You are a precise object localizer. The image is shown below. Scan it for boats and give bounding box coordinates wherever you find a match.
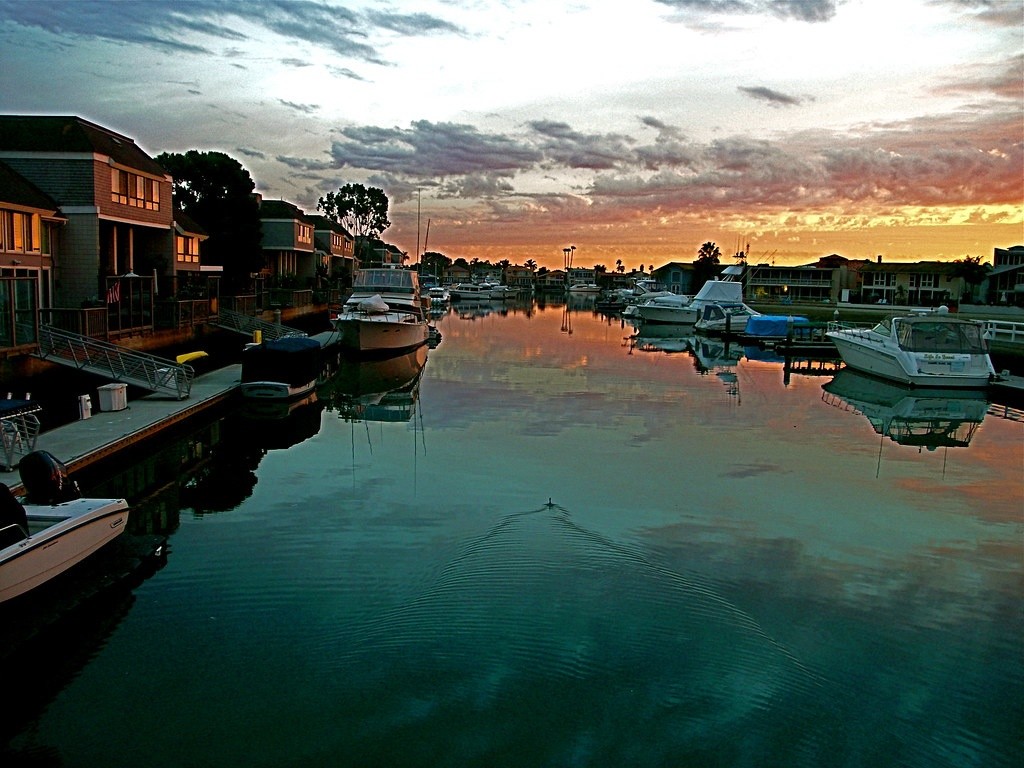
[239,336,325,399]
[820,366,992,480]
[567,283,603,292]
[427,286,451,306]
[329,268,432,353]
[449,280,523,300]
[822,309,998,387]
[320,343,430,422]
[629,322,745,373]
[693,301,764,334]
[594,274,744,324]
[0,449,131,606]
